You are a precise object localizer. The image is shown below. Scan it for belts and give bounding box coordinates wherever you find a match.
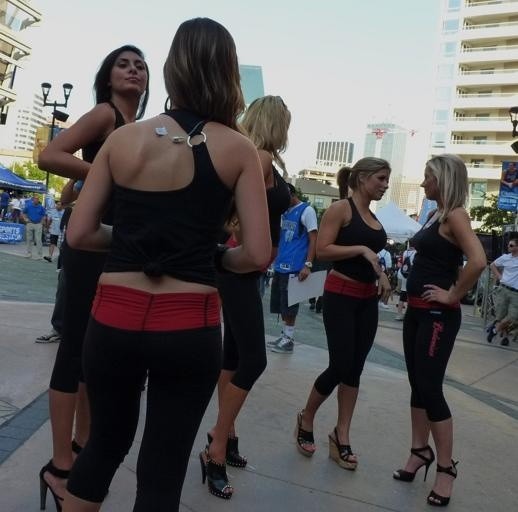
[501,283,518,293]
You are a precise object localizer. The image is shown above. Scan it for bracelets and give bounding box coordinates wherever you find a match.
[215,243,229,274]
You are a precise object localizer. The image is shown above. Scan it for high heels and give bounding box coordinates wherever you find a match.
[206,432,247,467]
[426,458,460,506]
[70,438,85,459]
[392,445,435,481]
[294,409,315,456]
[328,427,359,470]
[39,458,73,512]
[198,443,233,499]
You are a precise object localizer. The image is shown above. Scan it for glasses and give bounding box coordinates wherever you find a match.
[508,243,516,247]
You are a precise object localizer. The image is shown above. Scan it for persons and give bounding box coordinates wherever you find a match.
[293,157,393,470]
[226,232,418,322]
[486,238,518,347]
[265,184,318,353]
[499,162,518,194]
[199,96,291,500]
[36,45,151,510]
[34,178,82,345]
[390,154,489,505]
[0,188,67,264]
[60,16,272,511]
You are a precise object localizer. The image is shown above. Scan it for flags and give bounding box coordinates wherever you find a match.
[497,160,517,214]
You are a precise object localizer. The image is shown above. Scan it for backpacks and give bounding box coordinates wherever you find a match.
[401,250,413,278]
[378,250,388,273]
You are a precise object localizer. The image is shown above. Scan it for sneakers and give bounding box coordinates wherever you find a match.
[487,326,496,343]
[501,337,509,346]
[271,337,294,353]
[43,255,53,262]
[35,330,61,343]
[266,334,284,348]
[37,254,42,260]
[378,300,406,321]
[24,253,32,258]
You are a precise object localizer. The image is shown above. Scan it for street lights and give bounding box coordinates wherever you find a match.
[38,78,75,195]
